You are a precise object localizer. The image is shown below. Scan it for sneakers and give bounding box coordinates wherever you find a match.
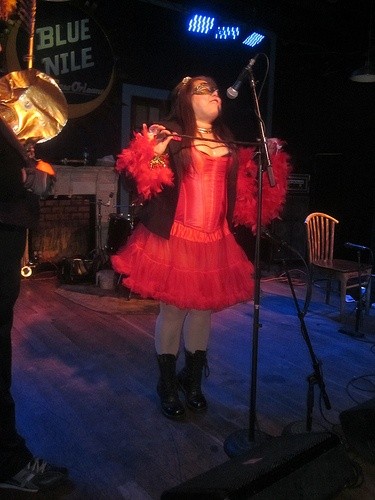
[0,454,69,493]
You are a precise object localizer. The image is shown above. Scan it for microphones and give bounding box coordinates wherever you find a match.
[226,53,262,99]
[345,242,369,251]
[262,226,287,247]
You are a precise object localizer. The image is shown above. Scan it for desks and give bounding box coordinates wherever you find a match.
[20,167,118,273]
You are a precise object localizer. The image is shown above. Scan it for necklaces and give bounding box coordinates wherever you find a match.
[196,126,213,134]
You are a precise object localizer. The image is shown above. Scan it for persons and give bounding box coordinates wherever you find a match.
[110,76,291,419]
[1,117,70,495]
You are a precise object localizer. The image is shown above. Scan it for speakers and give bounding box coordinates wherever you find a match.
[161,432,353,500]
[338,395,375,460]
[262,195,309,262]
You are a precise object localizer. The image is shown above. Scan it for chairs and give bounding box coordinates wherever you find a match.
[299,211,374,326]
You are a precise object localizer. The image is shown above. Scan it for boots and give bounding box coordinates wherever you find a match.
[179,348,210,412]
[155,353,186,418]
